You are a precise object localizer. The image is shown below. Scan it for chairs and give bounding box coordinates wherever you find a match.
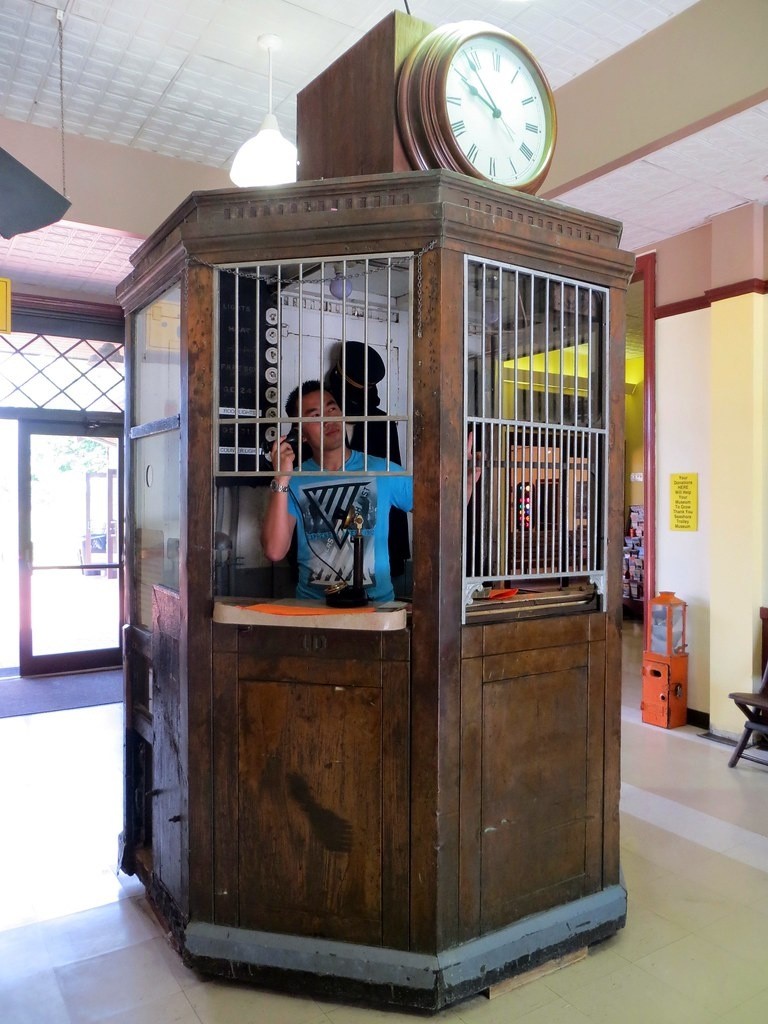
[727,663,768,770]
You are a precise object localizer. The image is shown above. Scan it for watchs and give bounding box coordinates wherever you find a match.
[270,480,288,492]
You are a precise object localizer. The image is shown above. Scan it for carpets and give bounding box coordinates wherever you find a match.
[0,665,124,718]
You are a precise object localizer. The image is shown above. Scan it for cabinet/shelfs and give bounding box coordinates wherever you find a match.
[621,504,644,623]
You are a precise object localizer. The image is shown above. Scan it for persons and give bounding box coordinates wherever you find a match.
[259,380,486,605]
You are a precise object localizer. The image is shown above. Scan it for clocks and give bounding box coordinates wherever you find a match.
[396,20,557,197]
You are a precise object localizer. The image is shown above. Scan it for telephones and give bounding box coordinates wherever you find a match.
[263,428,302,455]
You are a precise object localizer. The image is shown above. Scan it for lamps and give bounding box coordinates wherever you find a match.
[647,591,685,655]
[231,35,297,188]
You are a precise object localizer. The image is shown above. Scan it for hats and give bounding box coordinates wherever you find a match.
[330,342,385,403]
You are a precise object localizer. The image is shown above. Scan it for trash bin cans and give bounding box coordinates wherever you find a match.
[641,592,690,729]
